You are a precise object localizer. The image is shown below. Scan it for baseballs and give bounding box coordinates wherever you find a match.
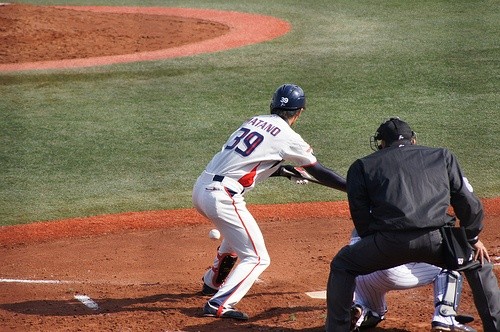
[208,228,221,240]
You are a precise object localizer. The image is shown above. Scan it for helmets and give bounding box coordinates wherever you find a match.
[271,84,305,110]
[374,118,416,142]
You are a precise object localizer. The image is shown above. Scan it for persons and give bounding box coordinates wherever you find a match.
[193,84,348,320]
[326,118,500,332]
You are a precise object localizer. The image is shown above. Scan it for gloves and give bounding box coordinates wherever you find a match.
[291,176,308,184]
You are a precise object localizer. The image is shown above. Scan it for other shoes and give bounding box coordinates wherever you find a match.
[431,320,476,332]
[202,301,249,320]
[351,304,363,332]
[202,287,219,296]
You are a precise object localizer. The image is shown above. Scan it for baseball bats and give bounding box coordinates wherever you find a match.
[279,168,347,192]
